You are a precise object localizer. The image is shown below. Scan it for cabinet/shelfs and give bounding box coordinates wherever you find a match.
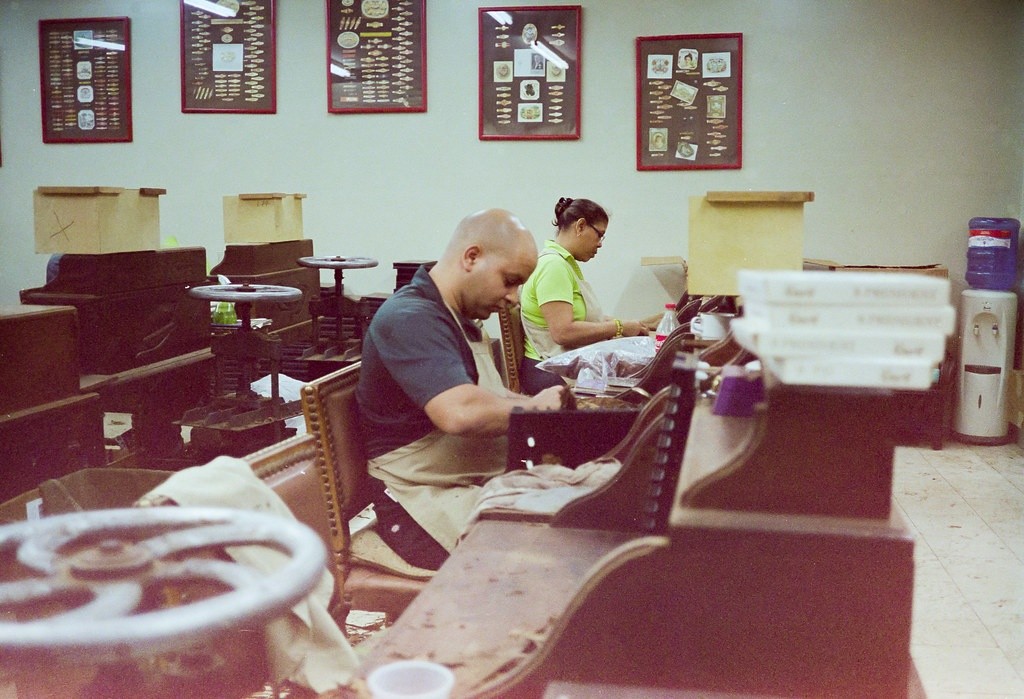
[358,291,930,698]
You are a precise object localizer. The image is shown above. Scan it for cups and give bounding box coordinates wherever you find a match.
[691,313,735,338]
[368,661,454,699]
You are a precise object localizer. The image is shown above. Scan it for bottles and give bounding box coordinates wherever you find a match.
[213,301,237,335]
[655,304,682,356]
[966,217,1020,290]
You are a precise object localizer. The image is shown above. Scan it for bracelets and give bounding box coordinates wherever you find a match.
[615,320,623,336]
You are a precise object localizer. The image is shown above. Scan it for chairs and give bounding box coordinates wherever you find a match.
[299,361,430,623]
[499,296,527,395]
[239,432,430,649]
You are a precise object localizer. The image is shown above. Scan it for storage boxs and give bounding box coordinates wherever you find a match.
[803,258,844,270]
[835,263,948,279]
[687,191,814,296]
[223,192,308,243]
[33,187,167,253]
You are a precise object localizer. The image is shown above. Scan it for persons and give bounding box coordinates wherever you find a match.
[355,210,564,572]
[520,197,650,396]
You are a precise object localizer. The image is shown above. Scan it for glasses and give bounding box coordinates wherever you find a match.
[586,222,606,244]
